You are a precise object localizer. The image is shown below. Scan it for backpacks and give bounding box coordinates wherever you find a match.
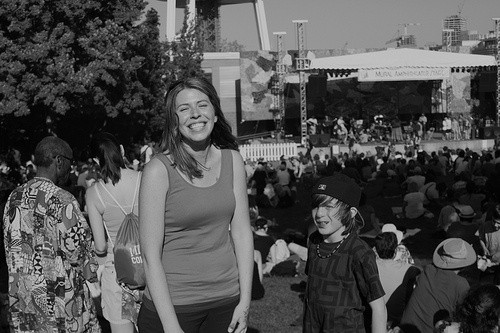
[96,168,146,290]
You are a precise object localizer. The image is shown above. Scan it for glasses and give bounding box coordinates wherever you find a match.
[52,153,76,166]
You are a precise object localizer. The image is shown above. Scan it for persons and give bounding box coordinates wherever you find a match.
[1,76,500,333]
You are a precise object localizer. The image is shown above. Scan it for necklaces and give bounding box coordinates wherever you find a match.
[316,230,349,258]
[34,176,54,185]
[194,148,210,170]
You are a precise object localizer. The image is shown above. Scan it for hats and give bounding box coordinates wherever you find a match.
[313,175,365,228]
[381,224,403,245]
[432,237,478,271]
